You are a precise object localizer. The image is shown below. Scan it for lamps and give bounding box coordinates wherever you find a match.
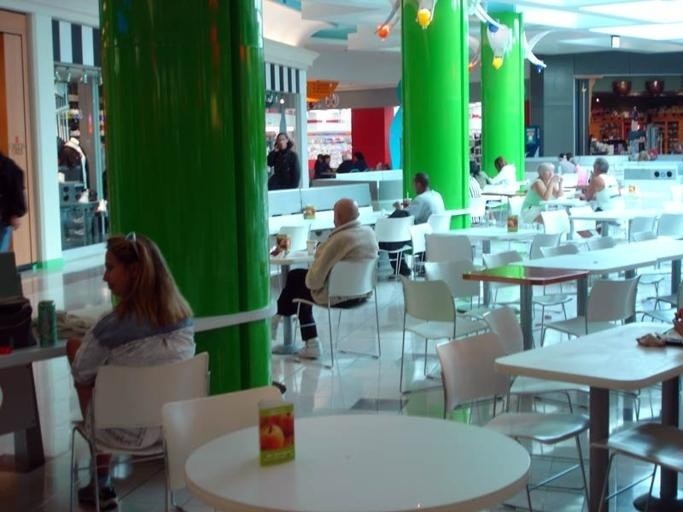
[611,35,621,49]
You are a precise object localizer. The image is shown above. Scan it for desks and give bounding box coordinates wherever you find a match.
[0,305,111,473]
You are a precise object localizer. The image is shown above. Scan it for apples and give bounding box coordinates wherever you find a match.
[260,424,285,450]
[276,412,295,436]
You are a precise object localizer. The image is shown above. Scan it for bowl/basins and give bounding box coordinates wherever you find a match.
[644,80,664,97]
[612,80,631,96]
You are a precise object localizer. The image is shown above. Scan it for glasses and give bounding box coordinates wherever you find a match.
[123,230,139,261]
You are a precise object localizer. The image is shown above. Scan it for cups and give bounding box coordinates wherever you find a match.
[306,239,318,256]
[403,198,411,208]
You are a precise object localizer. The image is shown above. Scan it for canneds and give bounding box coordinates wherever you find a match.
[38,300,58,349]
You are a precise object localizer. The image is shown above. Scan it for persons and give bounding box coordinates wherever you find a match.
[388,172,447,282]
[467,117,648,240]
[312,149,391,178]
[667,140,683,154]
[0,151,26,251]
[67,231,198,512]
[267,131,302,190]
[270,197,379,358]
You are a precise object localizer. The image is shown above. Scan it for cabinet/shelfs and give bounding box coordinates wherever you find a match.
[651,114,683,154]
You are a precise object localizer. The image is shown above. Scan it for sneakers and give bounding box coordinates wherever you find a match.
[294,340,323,359]
[382,268,413,281]
[77,482,118,511]
